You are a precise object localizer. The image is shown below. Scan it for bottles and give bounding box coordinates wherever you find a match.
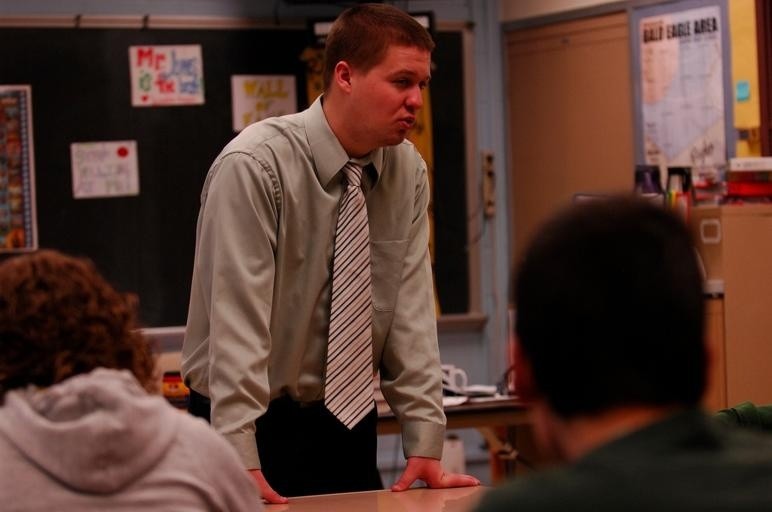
[666,164,696,207]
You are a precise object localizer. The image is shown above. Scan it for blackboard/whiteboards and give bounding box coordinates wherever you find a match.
[0,20,487,348]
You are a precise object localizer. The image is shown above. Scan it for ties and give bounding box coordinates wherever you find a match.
[324,163,375,431]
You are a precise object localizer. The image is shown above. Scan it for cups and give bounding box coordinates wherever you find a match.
[440,362,468,388]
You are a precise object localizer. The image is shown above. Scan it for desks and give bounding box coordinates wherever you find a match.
[252,390,551,512]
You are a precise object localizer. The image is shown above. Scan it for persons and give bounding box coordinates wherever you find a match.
[179,0,481,503]
[464,191,772,512]
[0,249,267,512]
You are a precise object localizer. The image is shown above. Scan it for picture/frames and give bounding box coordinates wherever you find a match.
[2,85,44,257]
